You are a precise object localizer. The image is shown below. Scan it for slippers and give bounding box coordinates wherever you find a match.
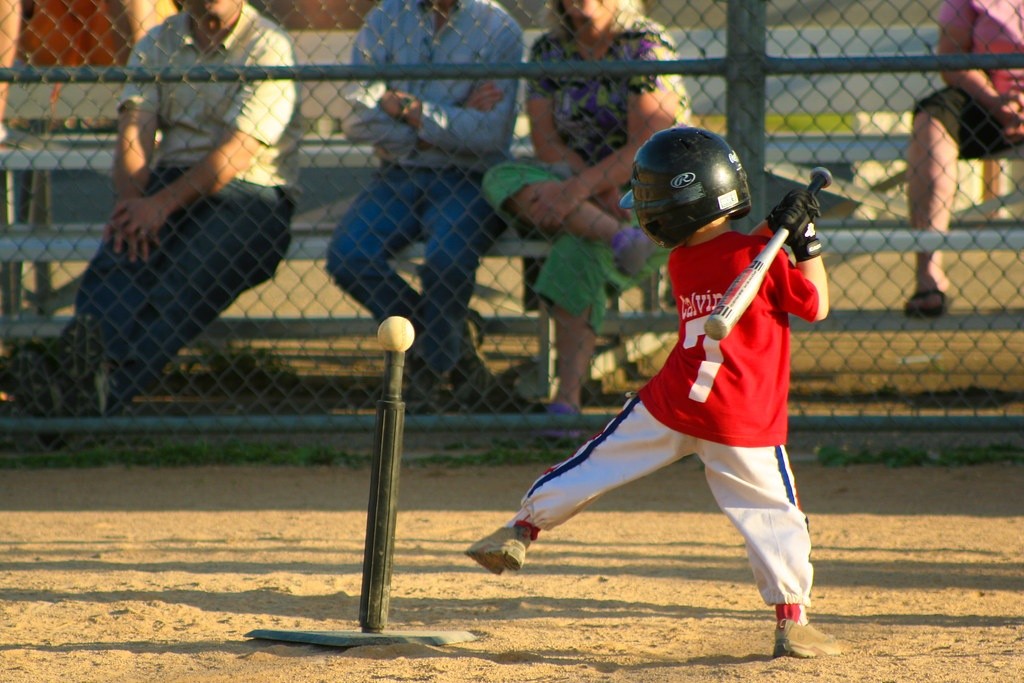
[904,290,946,317]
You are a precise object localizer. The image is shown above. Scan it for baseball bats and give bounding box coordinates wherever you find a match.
[703,166,835,342]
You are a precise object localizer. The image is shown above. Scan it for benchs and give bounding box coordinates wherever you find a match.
[0,24,1024,410]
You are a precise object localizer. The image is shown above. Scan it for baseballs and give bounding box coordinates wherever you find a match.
[376,315,415,352]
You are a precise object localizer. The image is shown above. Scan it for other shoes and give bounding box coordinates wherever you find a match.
[14,336,66,449]
[533,402,581,439]
[614,229,653,270]
[61,313,108,420]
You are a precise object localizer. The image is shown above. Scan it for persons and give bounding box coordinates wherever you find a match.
[0,0,297,448]
[326,0,857,660]
[904,0,1024,316]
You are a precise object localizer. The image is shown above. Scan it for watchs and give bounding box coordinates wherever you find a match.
[396,98,412,120]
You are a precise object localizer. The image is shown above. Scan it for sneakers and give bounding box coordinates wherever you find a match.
[466,526,533,574]
[772,618,844,659]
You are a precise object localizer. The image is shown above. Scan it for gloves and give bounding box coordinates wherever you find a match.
[766,187,824,262]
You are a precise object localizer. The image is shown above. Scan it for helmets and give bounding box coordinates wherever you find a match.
[620,126,750,248]
